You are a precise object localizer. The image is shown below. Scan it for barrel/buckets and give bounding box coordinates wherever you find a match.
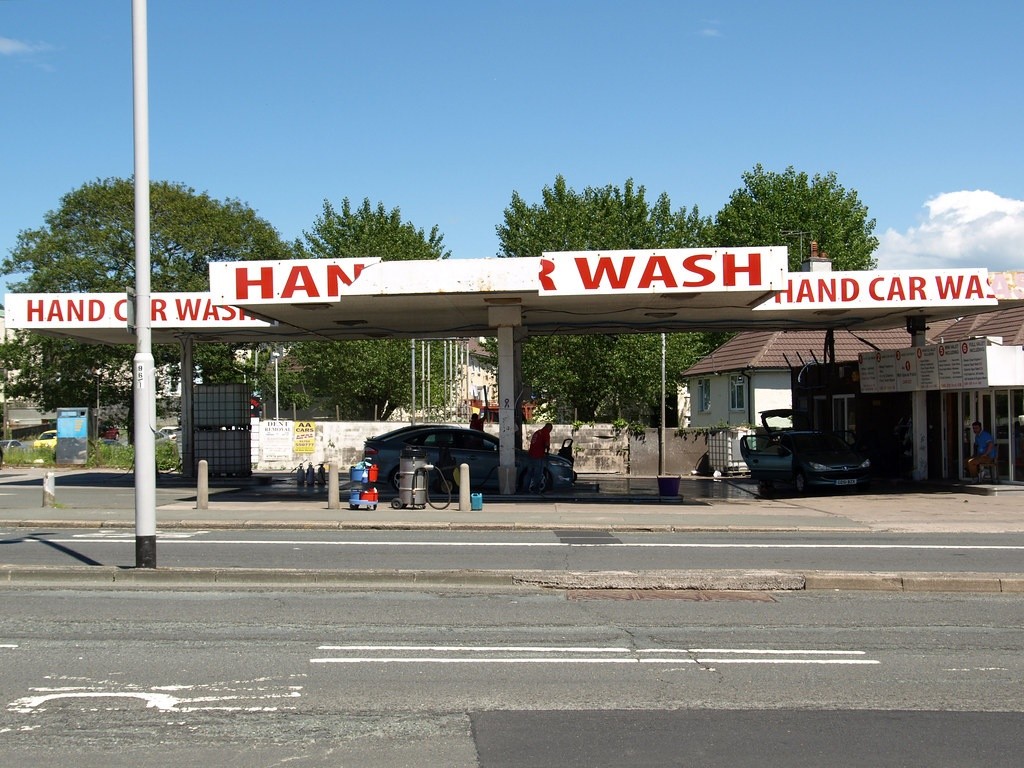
[656,476,681,496]
[471,493,482,510]
[350,468,379,501]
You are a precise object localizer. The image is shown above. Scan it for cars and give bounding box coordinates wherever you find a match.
[34,430,57,450]
[97,426,120,441]
[0,440,29,455]
[740,409,873,495]
[154,426,179,448]
[363,424,574,496]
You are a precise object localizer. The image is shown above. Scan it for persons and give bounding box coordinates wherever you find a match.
[523,423,552,494]
[968,421,997,485]
[470,406,489,431]
